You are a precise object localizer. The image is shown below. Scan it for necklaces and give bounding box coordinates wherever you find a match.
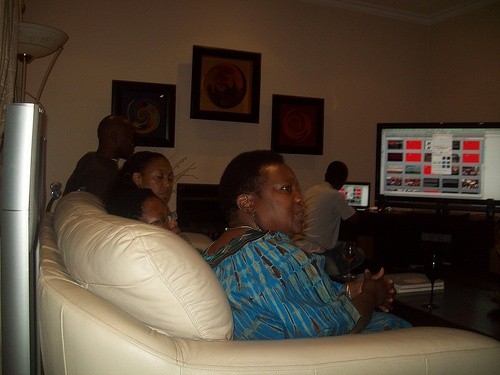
[224,225,262,233]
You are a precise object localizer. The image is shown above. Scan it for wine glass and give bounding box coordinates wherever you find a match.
[420,251,444,311]
[339,239,359,280]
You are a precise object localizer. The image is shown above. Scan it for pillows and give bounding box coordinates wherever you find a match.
[54,192,232,341]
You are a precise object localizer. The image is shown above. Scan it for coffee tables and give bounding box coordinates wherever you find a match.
[393,293,500,342]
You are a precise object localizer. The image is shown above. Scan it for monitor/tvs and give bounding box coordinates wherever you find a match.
[337,181,371,211]
[375,121,500,213]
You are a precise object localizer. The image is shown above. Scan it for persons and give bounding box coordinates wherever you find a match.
[198,149,414,340]
[60,114,414,340]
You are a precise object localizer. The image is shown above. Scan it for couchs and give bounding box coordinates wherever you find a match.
[35,191,500,375]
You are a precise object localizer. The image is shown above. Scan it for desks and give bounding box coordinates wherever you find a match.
[325,204,499,291]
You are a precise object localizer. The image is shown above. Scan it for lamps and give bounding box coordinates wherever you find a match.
[15,23,70,114]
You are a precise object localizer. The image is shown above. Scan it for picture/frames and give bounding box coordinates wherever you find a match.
[110,79,176,149]
[190,44,262,123]
[270,93,325,155]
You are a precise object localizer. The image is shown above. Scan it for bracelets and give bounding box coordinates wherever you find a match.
[345,283,353,301]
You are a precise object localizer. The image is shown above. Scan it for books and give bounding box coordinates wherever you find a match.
[385,274,444,293]
